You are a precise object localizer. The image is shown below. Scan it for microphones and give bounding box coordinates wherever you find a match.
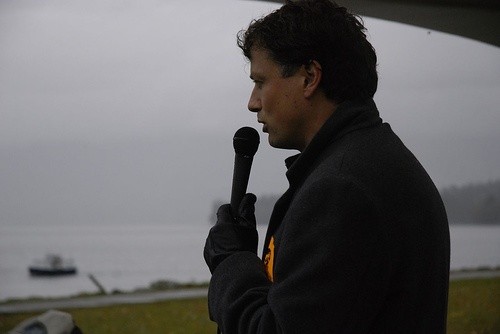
[230,126,261,219]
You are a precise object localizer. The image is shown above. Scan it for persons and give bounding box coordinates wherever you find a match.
[203,0,451,334]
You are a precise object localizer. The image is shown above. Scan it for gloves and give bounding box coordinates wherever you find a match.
[203,193,258,275]
